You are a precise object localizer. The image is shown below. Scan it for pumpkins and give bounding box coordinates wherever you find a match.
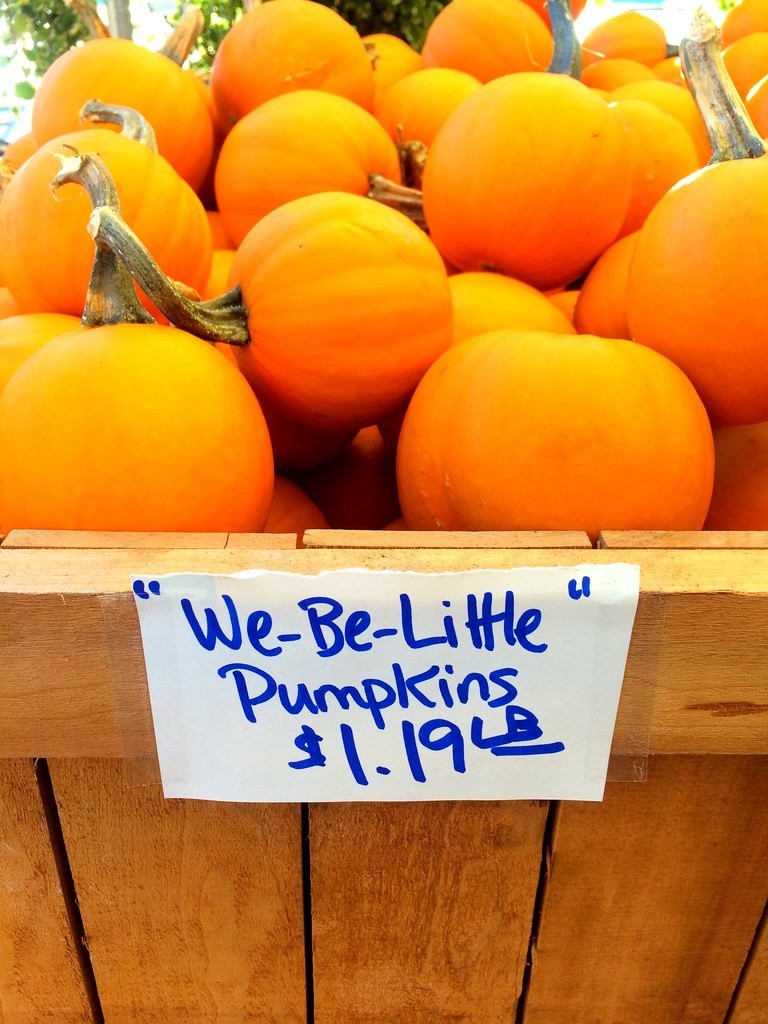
[0,0,768,546]
